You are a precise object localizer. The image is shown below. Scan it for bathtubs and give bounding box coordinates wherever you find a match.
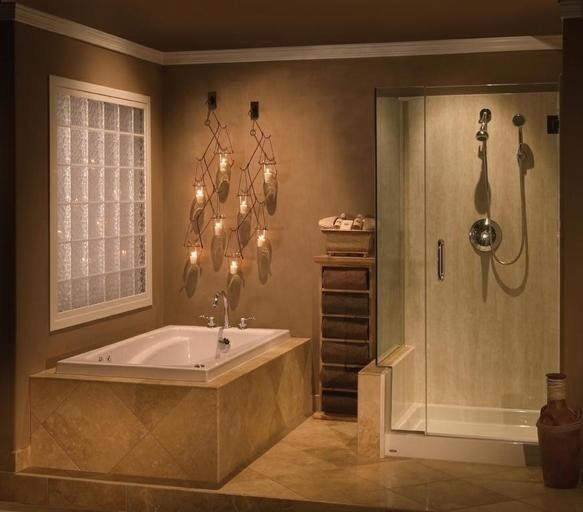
[22,324,315,488]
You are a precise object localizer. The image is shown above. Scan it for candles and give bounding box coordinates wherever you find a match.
[195,189,204,205]
[230,261,238,275]
[219,158,227,172]
[214,222,222,236]
[264,168,272,183]
[257,235,266,247]
[189,251,197,265]
[240,201,248,214]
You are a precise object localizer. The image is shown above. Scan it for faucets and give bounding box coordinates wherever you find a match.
[213,289,231,328]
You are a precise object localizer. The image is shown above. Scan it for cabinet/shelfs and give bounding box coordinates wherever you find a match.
[313,251,376,423]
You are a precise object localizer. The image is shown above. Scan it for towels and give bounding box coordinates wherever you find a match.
[319,269,368,416]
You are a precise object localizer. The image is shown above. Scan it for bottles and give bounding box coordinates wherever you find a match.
[351,213,363,231]
[333,212,345,229]
